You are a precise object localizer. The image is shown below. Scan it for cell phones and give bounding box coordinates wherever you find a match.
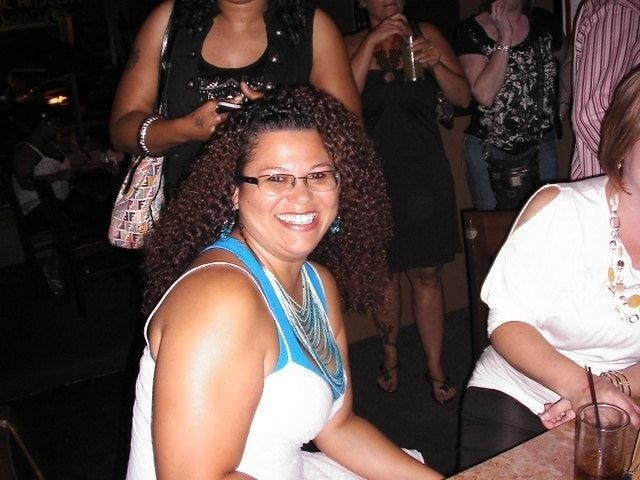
[216,100,243,117]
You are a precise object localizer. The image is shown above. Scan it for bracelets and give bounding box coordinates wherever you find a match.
[137,112,171,159]
[485,44,511,55]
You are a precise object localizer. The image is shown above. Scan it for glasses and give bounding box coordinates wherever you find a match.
[237,169,342,196]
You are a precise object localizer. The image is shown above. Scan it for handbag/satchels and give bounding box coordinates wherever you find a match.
[108,153,169,250]
[486,150,540,210]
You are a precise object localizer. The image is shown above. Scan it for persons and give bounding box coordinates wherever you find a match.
[448,0,576,213]
[107,0,372,210]
[118,81,451,480]
[312,0,474,404]
[563,0,640,183]
[433,57,640,476]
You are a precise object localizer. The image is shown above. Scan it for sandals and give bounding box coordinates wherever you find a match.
[377,361,400,392]
[428,375,455,406]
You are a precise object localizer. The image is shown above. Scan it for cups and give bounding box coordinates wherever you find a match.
[574,403,632,480]
[402,32,426,84]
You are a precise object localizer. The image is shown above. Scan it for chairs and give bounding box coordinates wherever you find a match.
[462,207,520,365]
[0,166,138,316]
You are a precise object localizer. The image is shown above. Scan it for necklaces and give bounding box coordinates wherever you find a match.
[602,186,640,326]
[368,33,405,83]
[235,223,350,396]
